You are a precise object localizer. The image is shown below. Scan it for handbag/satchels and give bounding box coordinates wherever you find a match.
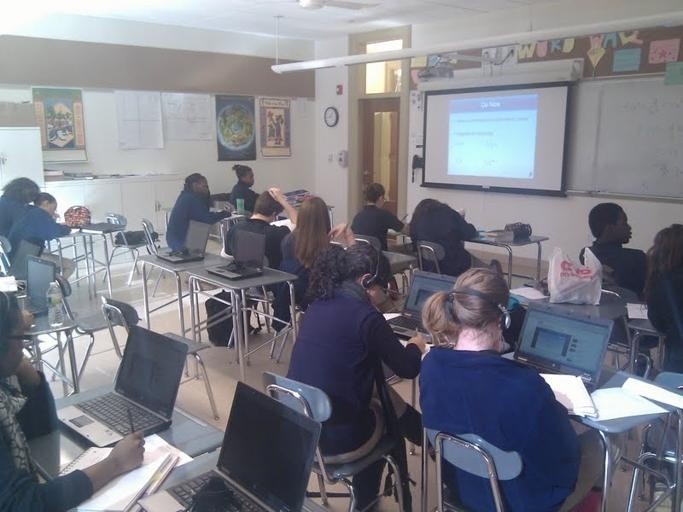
[64,205,91,227]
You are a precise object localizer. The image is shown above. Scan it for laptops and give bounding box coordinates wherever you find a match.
[15,254,57,316]
[513,300,607,384]
[157,219,211,263]
[386,270,458,343]
[205,229,267,280]
[135,380,325,512]
[56,326,191,448]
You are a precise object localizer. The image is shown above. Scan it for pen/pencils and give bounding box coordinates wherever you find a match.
[127,408,135,435]
[415,328,418,337]
[400,214,409,223]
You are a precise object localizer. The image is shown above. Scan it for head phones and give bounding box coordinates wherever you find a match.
[184,477,245,511]
[444,288,512,332]
[168,247,189,257]
[226,260,245,273]
[343,237,381,290]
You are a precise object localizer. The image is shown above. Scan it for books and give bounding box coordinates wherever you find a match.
[541,373,597,418]
[81,222,123,235]
[57,446,169,508]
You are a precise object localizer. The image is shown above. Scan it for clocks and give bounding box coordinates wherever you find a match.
[323,107,339,127]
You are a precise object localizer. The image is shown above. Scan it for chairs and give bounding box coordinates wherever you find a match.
[0,235,12,271]
[626,371,683,511]
[51,272,121,383]
[141,217,177,298]
[14,312,80,391]
[104,295,220,421]
[422,427,523,510]
[103,213,158,286]
[261,369,407,508]
[415,241,445,275]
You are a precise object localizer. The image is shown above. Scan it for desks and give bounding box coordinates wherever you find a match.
[57,223,126,298]
[137,247,233,339]
[186,258,299,381]
[567,366,668,511]
[471,231,550,287]
[357,248,419,274]
[627,319,666,377]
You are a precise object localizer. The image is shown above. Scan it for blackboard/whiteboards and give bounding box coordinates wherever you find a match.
[561,71,682,202]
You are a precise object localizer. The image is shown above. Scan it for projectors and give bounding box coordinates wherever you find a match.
[417,67,454,79]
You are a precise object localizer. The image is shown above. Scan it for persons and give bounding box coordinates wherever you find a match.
[580,203,661,380]
[1,292,145,510]
[646,224,683,373]
[225,187,297,335]
[412,198,503,277]
[230,164,287,221]
[166,173,235,253]
[286,242,436,512]
[0,178,40,237]
[272,196,358,332]
[419,268,624,511]
[352,183,411,300]
[7,192,76,279]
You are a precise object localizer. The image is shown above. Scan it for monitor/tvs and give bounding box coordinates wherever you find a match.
[7,238,42,281]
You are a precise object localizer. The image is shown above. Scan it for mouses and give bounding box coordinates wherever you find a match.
[18,282,25,291]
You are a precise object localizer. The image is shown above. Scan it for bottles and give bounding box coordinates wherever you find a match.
[47,282,64,327]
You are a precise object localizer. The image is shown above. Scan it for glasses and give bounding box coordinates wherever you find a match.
[4,334,33,345]
